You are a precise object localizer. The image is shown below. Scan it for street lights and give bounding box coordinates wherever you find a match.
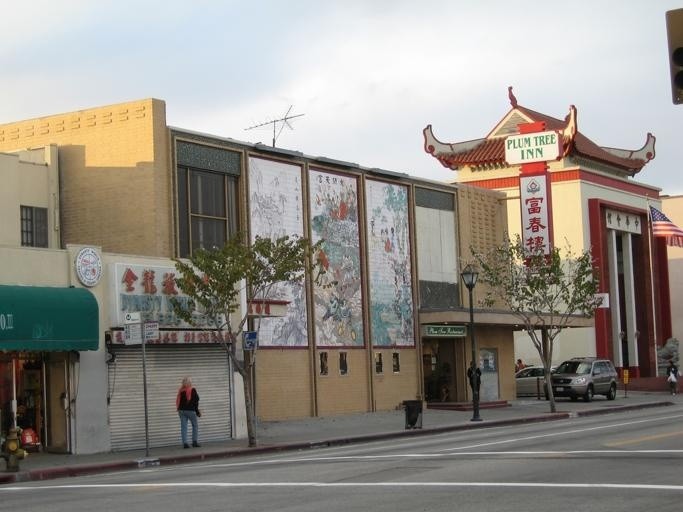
[459,263,483,421]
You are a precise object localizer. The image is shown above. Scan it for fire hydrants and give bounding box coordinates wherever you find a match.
[0,428,32,472]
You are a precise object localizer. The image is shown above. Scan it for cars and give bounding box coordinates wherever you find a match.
[515,356,618,402]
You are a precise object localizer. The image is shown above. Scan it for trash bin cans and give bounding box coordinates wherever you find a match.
[402,399,423,429]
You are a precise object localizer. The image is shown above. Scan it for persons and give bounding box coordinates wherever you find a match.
[466,360,481,402]
[665,360,678,396]
[174,376,202,448]
[516,359,524,370]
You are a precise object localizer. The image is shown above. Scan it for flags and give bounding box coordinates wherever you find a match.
[649,205,683,249]
